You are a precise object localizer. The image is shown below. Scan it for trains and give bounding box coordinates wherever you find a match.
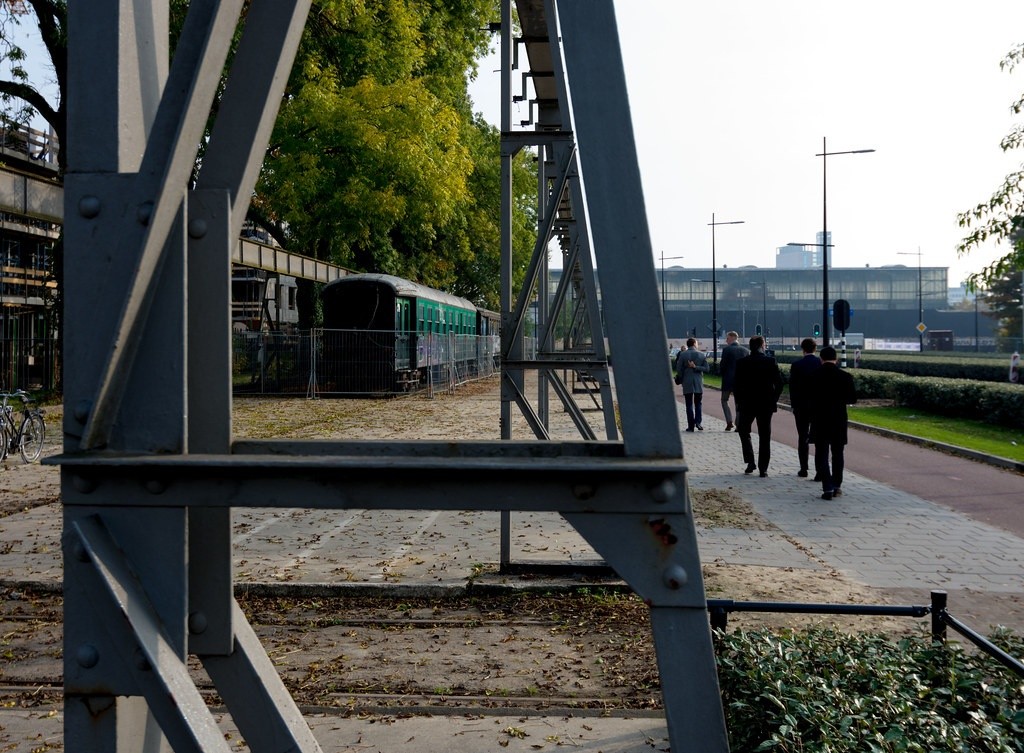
[317,273,502,391]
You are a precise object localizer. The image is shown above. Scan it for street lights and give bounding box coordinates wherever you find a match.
[658,252,684,317]
[689,279,701,340]
[694,213,746,364]
[787,136,878,345]
[748,271,767,350]
[897,246,931,352]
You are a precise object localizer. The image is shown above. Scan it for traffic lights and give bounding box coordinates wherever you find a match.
[691,326,696,338]
[814,322,821,337]
[755,324,762,336]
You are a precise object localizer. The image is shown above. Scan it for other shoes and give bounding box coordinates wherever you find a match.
[833,488,842,497]
[725,423,734,431]
[822,492,834,500]
[760,472,768,477]
[798,470,808,477]
[696,423,703,430]
[814,473,822,481]
[686,427,695,432]
[735,426,739,432]
[745,463,756,473]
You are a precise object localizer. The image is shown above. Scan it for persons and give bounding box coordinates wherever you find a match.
[674,338,709,433]
[804,347,857,500]
[675,345,687,368]
[717,331,749,432]
[789,341,824,479]
[733,336,785,479]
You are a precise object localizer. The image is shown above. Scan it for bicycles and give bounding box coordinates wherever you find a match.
[0,390,47,463]
